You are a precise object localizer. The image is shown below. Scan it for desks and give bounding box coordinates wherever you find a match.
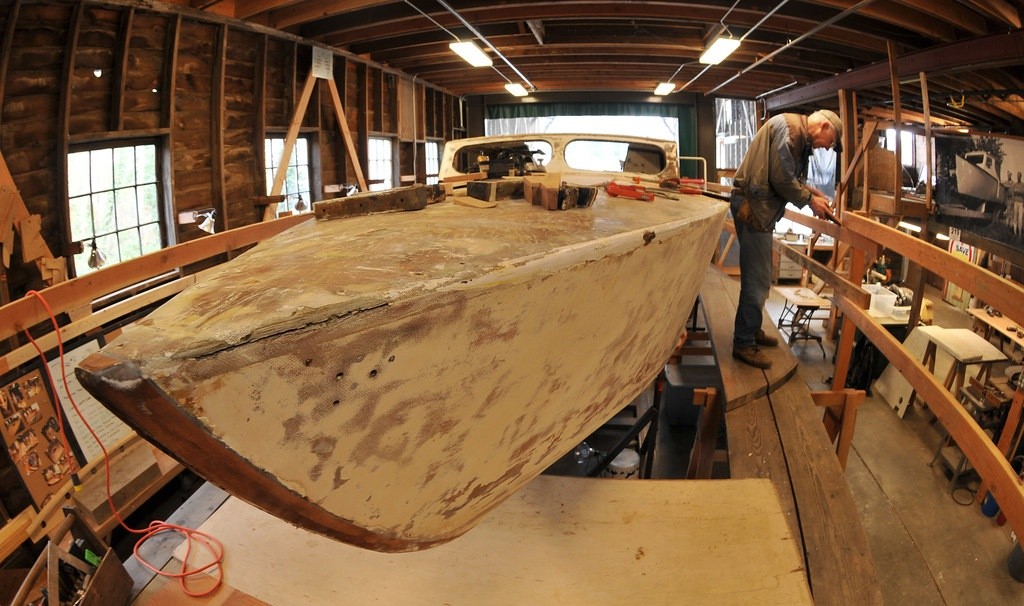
[772,288,827,355]
[966,307,1024,352]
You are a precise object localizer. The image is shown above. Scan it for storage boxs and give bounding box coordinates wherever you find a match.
[887,286,911,320]
[862,283,897,318]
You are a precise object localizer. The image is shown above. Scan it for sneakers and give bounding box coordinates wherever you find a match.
[754,330,778,346]
[732,343,772,368]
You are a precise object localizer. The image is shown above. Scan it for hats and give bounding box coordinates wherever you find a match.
[819,109,843,153]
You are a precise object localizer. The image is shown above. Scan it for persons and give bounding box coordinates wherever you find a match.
[730,110,844,369]
[1,382,77,480]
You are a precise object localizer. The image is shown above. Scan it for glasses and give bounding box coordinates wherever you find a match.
[830,127,837,148]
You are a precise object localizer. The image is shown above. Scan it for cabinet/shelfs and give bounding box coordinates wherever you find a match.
[779,245,805,280]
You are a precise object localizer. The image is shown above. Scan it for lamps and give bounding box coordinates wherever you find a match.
[178,207,215,235]
[293,195,309,210]
[324,183,360,196]
[699,36,742,65]
[505,81,529,97]
[653,81,676,96]
[87,240,106,269]
[450,38,493,68]
